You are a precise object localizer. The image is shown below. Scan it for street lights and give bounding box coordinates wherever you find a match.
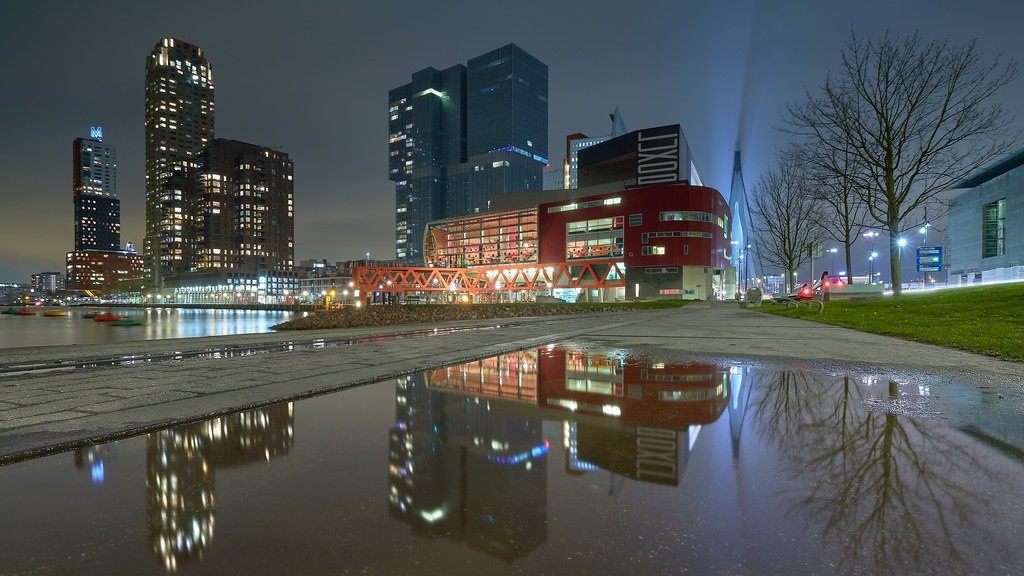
[781,272,787,294]
[763,275,767,294]
[771,275,777,294]
[793,271,798,286]
[863,230,878,285]
[755,277,760,288]
[921,220,931,290]
[743,243,752,292]
[827,247,837,277]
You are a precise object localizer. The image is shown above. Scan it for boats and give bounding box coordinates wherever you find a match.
[109,319,147,326]
[14,308,36,316]
[92,313,128,321]
[2,307,19,314]
[81,311,101,319]
[41,308,70,317]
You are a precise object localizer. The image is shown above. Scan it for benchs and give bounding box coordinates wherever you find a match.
[773,283,807,309]
[788,279,824,314]
